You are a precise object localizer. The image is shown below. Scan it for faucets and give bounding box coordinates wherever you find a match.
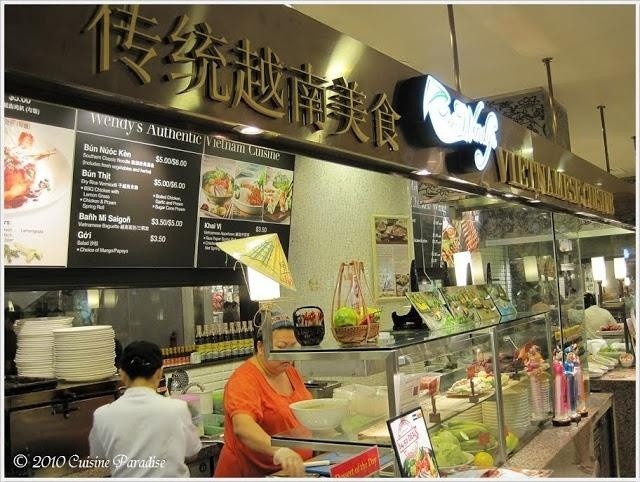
[181,382,204,394]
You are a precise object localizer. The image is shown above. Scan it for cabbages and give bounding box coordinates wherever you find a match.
[431,430,464,467]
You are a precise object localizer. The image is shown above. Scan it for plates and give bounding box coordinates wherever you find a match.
[303,382,328,388]
[450,376,550,437]
[401,362,424,375]
[234,176,262,214]
[12,315,117,384]
[587,356,618,379]
[436,440,499,471]
[4,138,67,214]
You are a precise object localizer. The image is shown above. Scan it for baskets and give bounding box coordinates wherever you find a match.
[332,261,380,344]
[293,306,325,345]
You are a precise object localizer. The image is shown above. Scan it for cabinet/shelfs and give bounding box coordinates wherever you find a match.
[264,308,558,479]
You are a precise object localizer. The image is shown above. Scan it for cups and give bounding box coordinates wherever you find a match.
[620,354,634,367]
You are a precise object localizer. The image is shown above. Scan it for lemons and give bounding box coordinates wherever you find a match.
[474,452,494,468]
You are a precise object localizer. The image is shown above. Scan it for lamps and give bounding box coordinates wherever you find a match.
[613,256,628,297]
[213,232,297,359]
[522,255,540,285]
[451,250,475,288]
[468,249,493,284]
[590,255,607,307]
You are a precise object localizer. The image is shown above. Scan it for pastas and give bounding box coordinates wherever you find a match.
[376,221,407,242]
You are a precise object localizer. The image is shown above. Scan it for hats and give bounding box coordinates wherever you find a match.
[122,342,164,368]
[253,304,294,339]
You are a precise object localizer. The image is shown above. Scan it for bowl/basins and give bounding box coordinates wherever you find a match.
[202,414,225,435]
[396,274,409,286]
[448,227,457,239]
[386,225,407,240]
[287,398,349,432]
[201,171,235,206]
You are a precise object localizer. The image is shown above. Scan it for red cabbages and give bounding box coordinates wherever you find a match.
[333,307,358,328]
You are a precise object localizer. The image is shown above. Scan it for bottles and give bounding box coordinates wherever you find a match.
[161,345,197,368]
[553,358,586,423]
[194,320,254,363]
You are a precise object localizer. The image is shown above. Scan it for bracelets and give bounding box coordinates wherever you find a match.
[273,447,288,466]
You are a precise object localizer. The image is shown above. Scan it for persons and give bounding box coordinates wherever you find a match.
[530,294,549,311]
[88,340,204,477]
[212,311,342,477]
[565,293,618,340]
